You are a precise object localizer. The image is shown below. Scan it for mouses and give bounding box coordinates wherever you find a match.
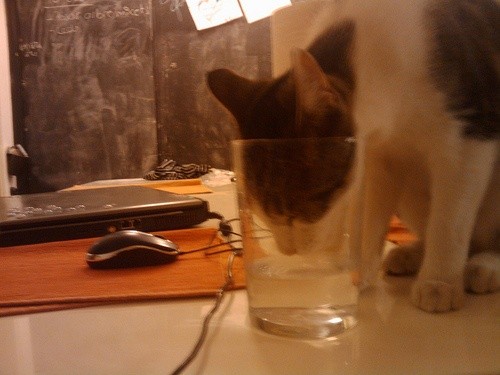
[87,229,180,268]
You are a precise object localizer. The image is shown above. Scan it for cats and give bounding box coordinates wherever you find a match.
[205,0,500,312]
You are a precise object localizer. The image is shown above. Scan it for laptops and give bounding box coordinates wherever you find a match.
[0,184,211,248]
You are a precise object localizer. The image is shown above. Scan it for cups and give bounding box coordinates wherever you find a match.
[229,133,371,342]
